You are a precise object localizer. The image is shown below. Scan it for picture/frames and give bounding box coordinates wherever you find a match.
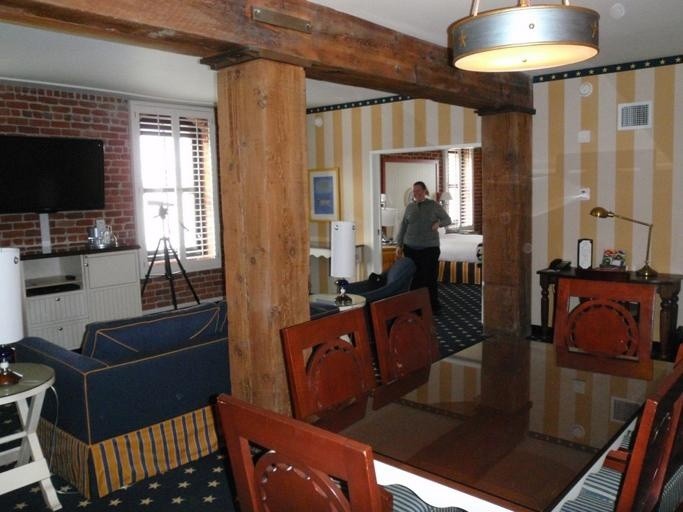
[307,166,341,223]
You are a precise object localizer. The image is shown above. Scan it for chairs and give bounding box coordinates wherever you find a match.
[552,361,683,512]
[606,343,683,512]
[371,286,441,386]
[552,277,655,360]
[280,306,376,421]
[215,391,462,512]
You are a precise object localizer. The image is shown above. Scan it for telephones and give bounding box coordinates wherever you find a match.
[549,258,571,270]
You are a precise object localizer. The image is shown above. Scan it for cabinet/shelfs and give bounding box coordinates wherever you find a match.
[20,244,143,351]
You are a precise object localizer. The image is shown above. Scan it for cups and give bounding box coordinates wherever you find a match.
[88,237,95,250]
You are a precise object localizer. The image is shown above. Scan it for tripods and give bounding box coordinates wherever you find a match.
[141,204,203,311]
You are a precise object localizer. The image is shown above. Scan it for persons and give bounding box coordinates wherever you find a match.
[395,181,452,316]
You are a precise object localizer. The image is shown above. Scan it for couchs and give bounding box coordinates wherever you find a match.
[346,256,416,302]
[14,298,340,500]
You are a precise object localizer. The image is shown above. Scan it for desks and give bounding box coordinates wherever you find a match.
[382,240,397,273]
[536,265,683,362]
[301,333,676,512]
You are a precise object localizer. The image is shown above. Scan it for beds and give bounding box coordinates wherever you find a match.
[438,226,483,286]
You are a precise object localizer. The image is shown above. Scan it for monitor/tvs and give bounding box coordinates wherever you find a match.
[0,132,105,216]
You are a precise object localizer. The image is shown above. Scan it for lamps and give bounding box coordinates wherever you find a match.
[329,220,356,307]
[439,191,452,208]
[381,202,397,228]
[0,247,26,386]
[448,0,601,75]
[589,206,658,278]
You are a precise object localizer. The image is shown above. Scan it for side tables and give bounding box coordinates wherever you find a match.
[0,361,63,511]
